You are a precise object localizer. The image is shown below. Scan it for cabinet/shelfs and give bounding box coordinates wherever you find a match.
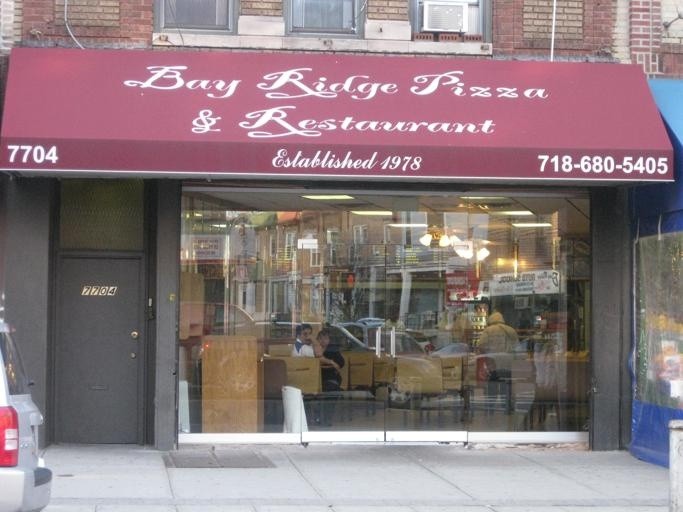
[199,334,264,432]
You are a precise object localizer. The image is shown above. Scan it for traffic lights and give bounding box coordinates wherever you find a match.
[342,272,355,290]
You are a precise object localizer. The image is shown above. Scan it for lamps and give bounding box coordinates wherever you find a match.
[416,212,462,246]
[451,211,490,261]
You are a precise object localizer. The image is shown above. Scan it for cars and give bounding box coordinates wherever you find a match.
[473,335,552,383]
[336,317,430,359]
[192,321,443,409]
[428,341,483,398]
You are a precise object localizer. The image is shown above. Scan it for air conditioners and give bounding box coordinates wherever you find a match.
[417,1,468,41]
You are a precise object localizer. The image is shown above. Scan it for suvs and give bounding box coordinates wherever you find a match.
[0,321,52,512]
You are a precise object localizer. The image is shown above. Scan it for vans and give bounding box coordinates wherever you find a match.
[178,300,252,373]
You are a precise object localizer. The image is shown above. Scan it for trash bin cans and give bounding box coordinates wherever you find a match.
[667,419,683,512]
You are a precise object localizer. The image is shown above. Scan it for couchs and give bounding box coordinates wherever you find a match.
[264,342,592,430]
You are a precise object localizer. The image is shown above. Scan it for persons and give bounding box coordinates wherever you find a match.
[478,311,520,416]
[382,306,404,352]
[290,324,323,358]
[313,330,345,426]
